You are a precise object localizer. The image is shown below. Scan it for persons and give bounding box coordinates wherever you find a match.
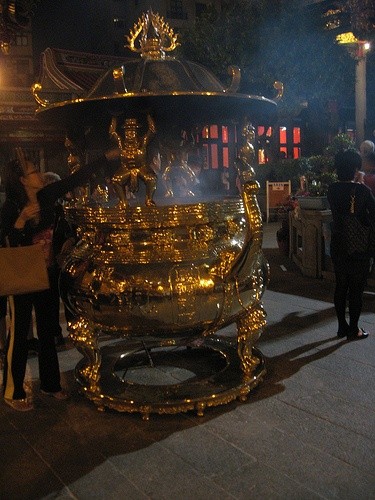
[328,148,375,341]
[359,140,375,197]
[108,105,198,210]
[231,115,256,199]
[0,150,231,413]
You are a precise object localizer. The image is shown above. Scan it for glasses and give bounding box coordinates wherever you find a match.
[27,168,39,175]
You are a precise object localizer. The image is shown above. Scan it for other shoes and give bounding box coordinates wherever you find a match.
[4,398,33,411]
[40,390,68,400]
[347,328,368,340]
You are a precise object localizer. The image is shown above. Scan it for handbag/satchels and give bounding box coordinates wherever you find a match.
[0,236,50,297]
[340,182,375,258]
